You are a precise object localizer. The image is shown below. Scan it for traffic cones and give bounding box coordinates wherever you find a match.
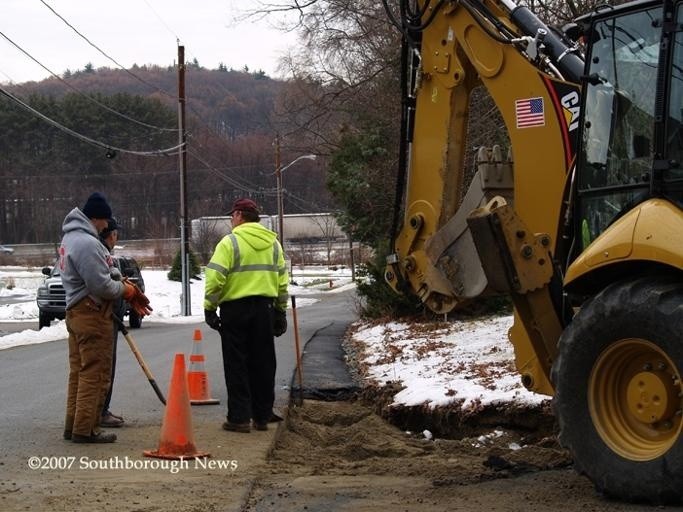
[187,330,220,404]
[143,354,210,460]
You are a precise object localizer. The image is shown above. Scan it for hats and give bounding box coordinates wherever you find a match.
[81,191,114,221]
[223,197,261,216]
[101,215,124,235]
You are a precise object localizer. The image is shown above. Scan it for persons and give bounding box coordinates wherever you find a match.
[57,192,137,445]
[101,219,127,427]
[202,198,289,433]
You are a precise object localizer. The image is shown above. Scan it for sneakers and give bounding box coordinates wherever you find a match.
[253,416,268,431]
[96,409,125,428]
[222,417,253,434]
[62,425,118,444]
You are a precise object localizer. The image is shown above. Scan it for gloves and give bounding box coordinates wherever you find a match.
[271,305,289,338]
[201,307,223,331]
[122,278,154,316]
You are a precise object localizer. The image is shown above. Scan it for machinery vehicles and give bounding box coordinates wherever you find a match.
[382,0,683,504]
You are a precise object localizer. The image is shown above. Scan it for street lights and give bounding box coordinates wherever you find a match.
[273,134,317,251]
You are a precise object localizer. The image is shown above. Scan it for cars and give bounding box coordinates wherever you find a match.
[36,256,145,329]
